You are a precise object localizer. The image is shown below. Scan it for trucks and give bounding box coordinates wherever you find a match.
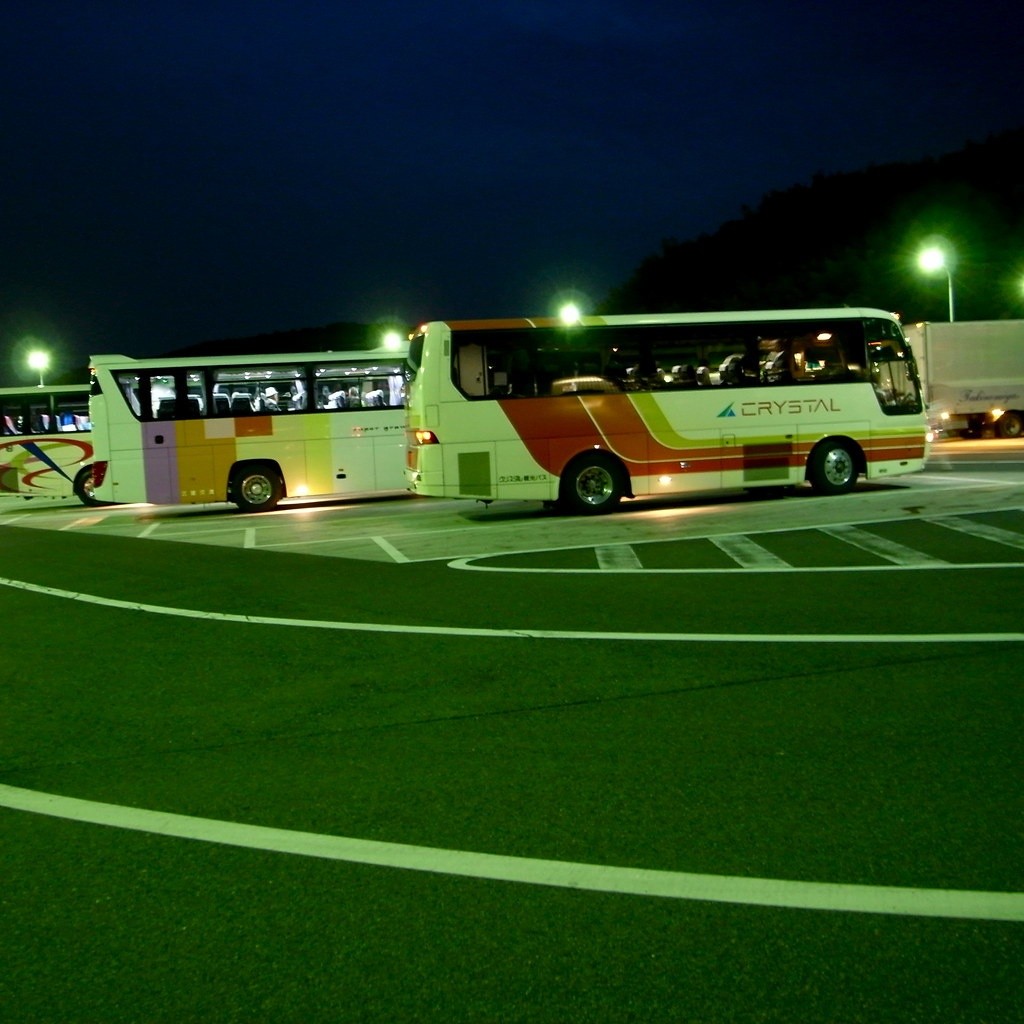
[870,320,1024,442]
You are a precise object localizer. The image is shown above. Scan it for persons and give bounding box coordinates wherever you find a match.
[726,351,798,383]
[249,381,408,411]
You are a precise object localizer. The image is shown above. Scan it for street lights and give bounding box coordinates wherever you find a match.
[921,249,954,321]
[28,349,49,388]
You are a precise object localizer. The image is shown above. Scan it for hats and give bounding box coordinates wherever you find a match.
[265,386,278,399]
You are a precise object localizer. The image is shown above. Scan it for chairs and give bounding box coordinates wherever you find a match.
[3,414,91,435]
[157,389,382,418]
[624,351,807,388]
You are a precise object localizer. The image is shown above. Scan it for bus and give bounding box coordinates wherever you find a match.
[86,346,477,513]
[401,307,930,516]
[0,381,176,503]
